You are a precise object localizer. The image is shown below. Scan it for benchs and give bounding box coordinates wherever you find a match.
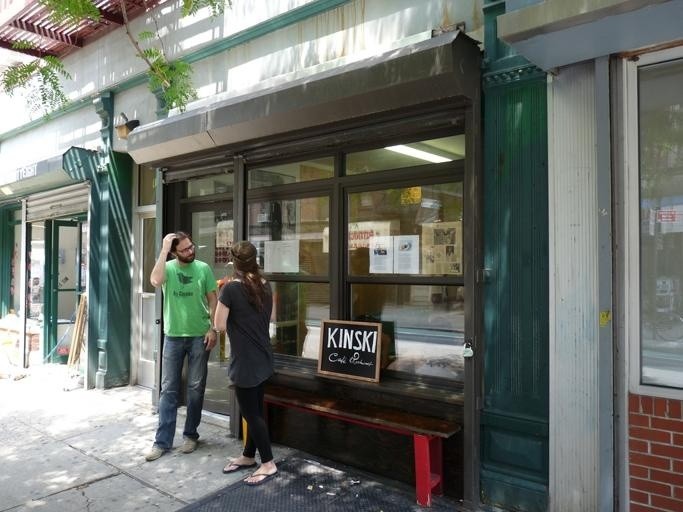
[223,383,462,506]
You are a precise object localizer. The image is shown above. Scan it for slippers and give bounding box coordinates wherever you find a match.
[223,461,257,473]
[244,471,279,486]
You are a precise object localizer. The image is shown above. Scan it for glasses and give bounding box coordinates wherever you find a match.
[176,244,194,254]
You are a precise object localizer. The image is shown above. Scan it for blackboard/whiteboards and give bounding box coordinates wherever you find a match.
[317,320,383,383]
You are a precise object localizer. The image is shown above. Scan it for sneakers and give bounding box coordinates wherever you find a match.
[181,438,197,453]
[146,447,165,461]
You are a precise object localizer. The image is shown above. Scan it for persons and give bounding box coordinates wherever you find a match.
[146,231,217,461]
[214,241,278,485]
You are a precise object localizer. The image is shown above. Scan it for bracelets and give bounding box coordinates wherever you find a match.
[211,327,220,337]
